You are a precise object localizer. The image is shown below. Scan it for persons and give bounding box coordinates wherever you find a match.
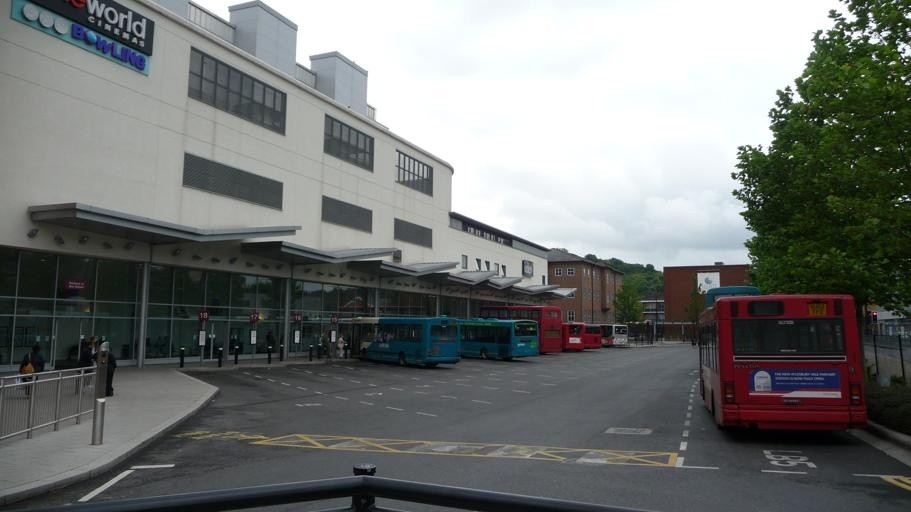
[76,342,97,394]
[94,341,117,397]
[338,335,347,359]
[19,344,46,397]
[319,329,333,360]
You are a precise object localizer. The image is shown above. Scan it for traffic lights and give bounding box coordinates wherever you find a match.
[873,311,877,323]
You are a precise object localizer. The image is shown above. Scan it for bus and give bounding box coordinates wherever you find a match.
[351,305,628,368]
[700,286,868,431]
